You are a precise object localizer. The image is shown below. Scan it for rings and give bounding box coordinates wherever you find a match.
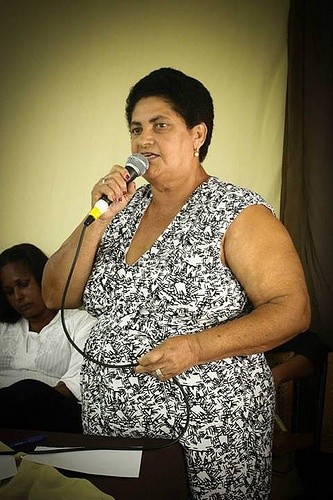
[156,369,164,379]
[103,177,107,184]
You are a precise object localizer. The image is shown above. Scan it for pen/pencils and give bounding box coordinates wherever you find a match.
[10,433,47,448]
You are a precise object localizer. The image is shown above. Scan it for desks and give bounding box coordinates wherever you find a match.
[0,429,193,500]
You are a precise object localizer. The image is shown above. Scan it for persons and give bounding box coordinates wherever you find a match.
[0,242,98,443]
[271,329,327,385]
[41,66,311,500]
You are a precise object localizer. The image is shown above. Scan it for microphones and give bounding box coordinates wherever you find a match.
[84,153,148,226]
